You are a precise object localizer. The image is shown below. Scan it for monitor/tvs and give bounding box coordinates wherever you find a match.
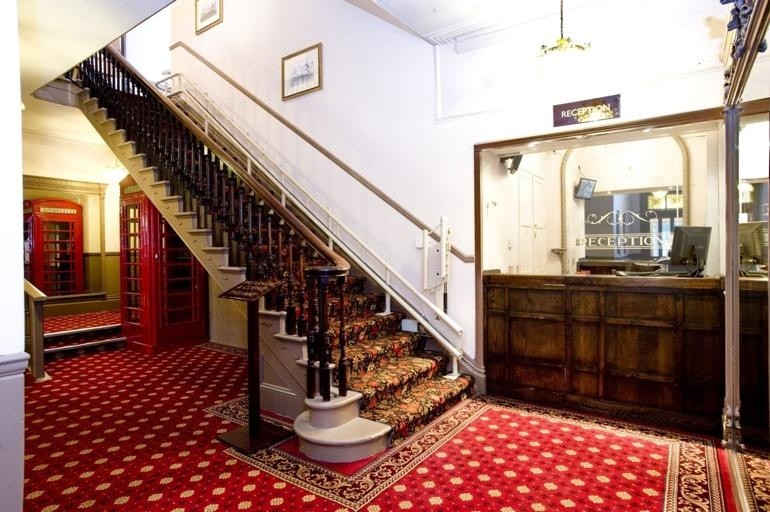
[670,226,712,278]
[738,222,768,276]
[574,178,597,200]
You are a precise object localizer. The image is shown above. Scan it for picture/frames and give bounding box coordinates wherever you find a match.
[280,42,323,101]
[194,0,223,36]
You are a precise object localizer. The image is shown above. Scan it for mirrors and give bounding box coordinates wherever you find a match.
[736,122,768,266]
[560,133,690,271]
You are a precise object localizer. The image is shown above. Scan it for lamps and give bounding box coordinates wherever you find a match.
[539,0,592,58]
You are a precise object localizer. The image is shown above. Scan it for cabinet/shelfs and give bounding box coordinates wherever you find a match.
[480,272,770,456]
